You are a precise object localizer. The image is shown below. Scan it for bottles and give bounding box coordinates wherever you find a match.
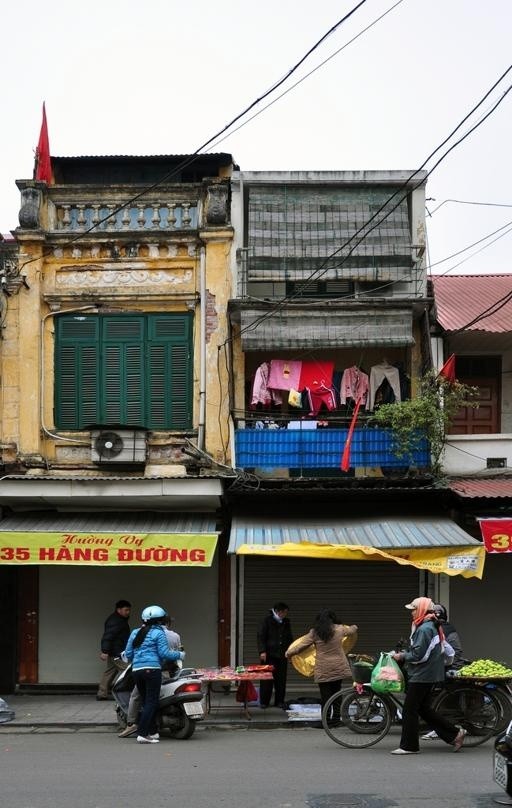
[405,597,435,610]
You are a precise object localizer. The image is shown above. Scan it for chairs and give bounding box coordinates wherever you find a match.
[260,704,268,708]
[316,718,340,729]
[96,696,115,701]
[422,731,439,740]
[137,733,160,743]
[275,702,288,708]
[118,723,138,738]
[391,748,421,755]
[453,729,468,753]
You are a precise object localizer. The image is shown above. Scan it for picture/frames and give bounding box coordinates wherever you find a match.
[193,665,274,720]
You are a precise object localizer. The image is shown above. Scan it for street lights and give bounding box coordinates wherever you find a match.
[443,640,455,666]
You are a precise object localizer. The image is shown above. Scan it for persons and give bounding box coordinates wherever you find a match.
[117,611,184,737]
[96,598,133,701]
[391,596,467,755]
[257,601,295,708]
[122,606,187,744]
[285,607,359,730]
[421,604,462,740]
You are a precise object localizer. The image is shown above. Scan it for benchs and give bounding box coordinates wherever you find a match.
[440,351,458,386]
[34,102,57,185]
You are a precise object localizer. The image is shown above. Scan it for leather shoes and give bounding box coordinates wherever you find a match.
[179,651,185,661]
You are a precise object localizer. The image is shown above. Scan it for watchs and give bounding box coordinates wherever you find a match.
[110,647,204,740]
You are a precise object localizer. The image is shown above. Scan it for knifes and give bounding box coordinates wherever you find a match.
[90,429,147,467]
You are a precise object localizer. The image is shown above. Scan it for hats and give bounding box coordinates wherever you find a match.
[495,717,512,804]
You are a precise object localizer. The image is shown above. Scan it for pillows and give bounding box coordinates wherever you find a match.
[434,605,447,621]
[141,606,166,621]
